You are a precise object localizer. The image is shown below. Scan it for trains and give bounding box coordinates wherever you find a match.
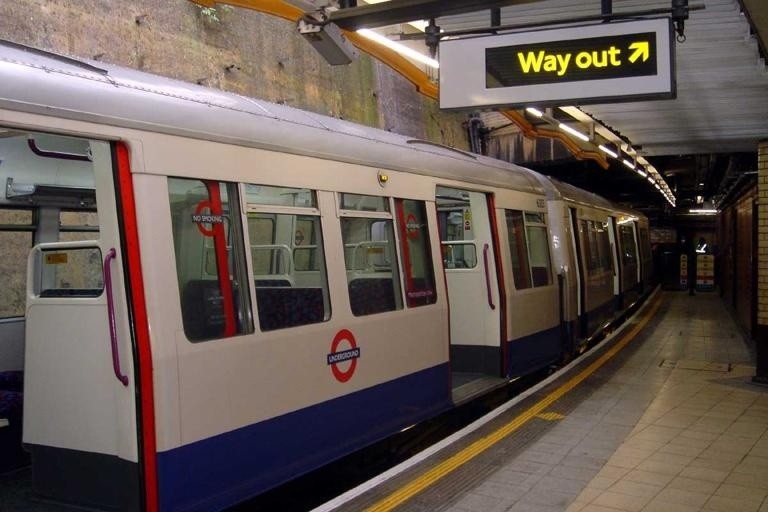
[0,38,655,511]
